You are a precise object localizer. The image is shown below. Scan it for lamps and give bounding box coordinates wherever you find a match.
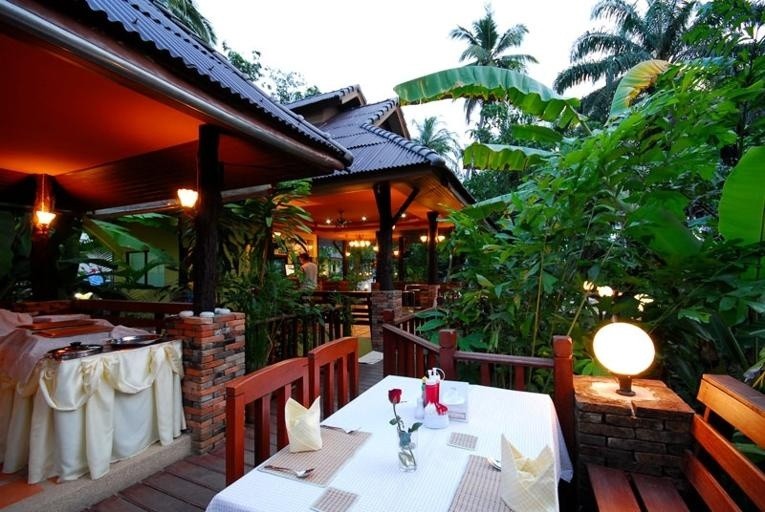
[592,322,657,398]
[32,168,57,242]
[335,209,352,231]
[420,229,428,243]
[176,187,200,217]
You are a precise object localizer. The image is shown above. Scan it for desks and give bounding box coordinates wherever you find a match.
[1,316,188,483]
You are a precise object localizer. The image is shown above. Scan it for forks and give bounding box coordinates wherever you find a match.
[320,423,357,435]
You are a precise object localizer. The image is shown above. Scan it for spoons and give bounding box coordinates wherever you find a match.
[488,455,502,471]
[263,464,315,478]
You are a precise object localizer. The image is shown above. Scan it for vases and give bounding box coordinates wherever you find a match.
[392,420,418,473]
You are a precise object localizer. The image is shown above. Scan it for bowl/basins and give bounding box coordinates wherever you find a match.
[423,413,448,428]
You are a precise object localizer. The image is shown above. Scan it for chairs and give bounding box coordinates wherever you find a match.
[404,282,441,312]
[308,335,359,423]
[224,357,312,487]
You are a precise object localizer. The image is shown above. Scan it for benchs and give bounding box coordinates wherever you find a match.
[587,374,765,510]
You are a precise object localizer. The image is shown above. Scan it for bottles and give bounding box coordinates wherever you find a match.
[396,424,420,472]
[426,377,436,404]
[434,375,441,405]
[415,398,425,421]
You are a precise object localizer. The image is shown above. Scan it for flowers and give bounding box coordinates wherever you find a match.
[387,389,423,465]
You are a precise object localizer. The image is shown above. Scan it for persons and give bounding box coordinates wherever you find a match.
[295,253,318,291]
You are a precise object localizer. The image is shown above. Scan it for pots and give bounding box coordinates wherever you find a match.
[109,334,166,349]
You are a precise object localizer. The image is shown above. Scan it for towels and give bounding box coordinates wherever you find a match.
[500,434,561,512]
[285,394,321,452]
